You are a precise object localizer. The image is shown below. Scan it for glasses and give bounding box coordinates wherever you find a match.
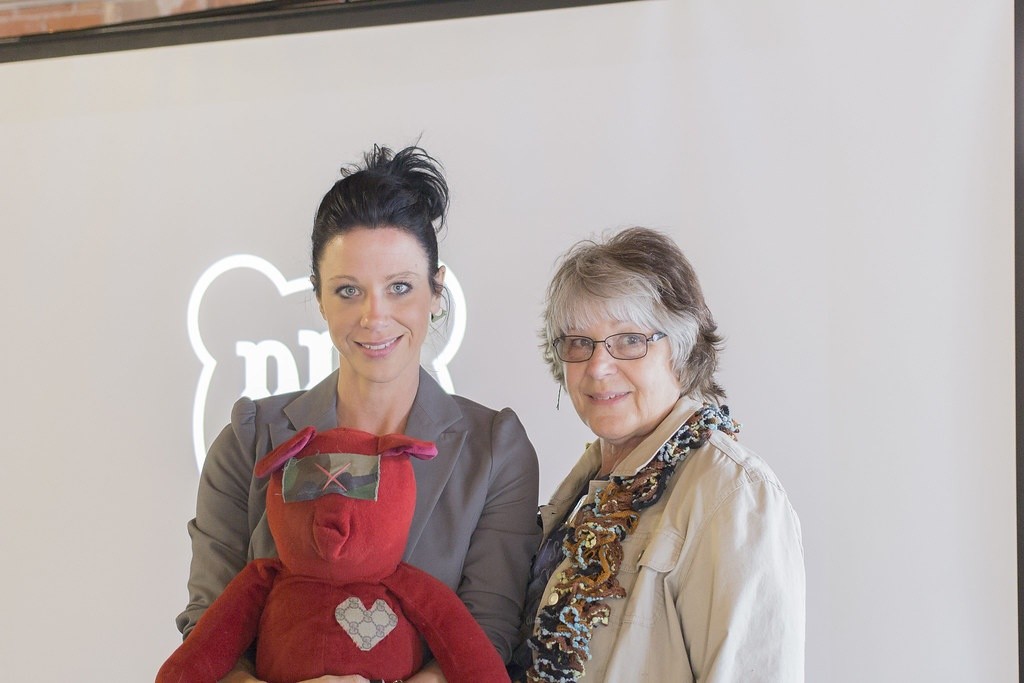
[553,332,668,363]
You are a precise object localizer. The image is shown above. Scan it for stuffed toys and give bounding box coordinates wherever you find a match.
[155,427,511,683]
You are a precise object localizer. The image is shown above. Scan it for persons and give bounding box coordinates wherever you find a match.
[501,228,805,683]
[175,148,538,683]
